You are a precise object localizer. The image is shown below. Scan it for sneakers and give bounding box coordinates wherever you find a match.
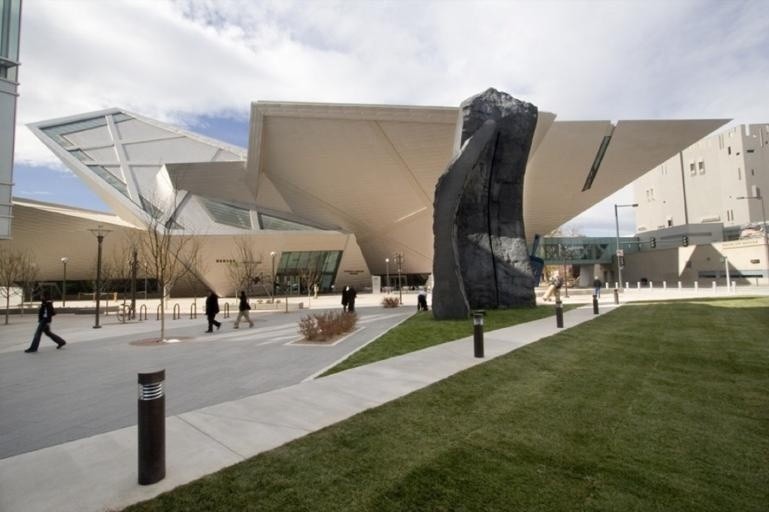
[24,348,37,352]
[57,341,65,350]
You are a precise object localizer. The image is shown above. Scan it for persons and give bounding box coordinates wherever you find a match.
[330,282,337,293]
[205,288,221,333]
[417,290,428,311]
[593,276,602,299]
[232,290,254,329]
[546,275,562,300]
[412,284,416,290]
[346,284,357,312]
[341,285,348,312]
[24,290,67,353]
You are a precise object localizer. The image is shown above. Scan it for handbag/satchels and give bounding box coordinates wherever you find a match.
[246,303,251,310]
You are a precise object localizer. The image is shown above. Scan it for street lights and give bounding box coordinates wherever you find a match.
[88,226,113,328]
[615,204,638,288]
[61,257,69,306]
[270,252,277,303]
[145,263,147,299]
[736,197,769,261]
[385,259,389,294]
